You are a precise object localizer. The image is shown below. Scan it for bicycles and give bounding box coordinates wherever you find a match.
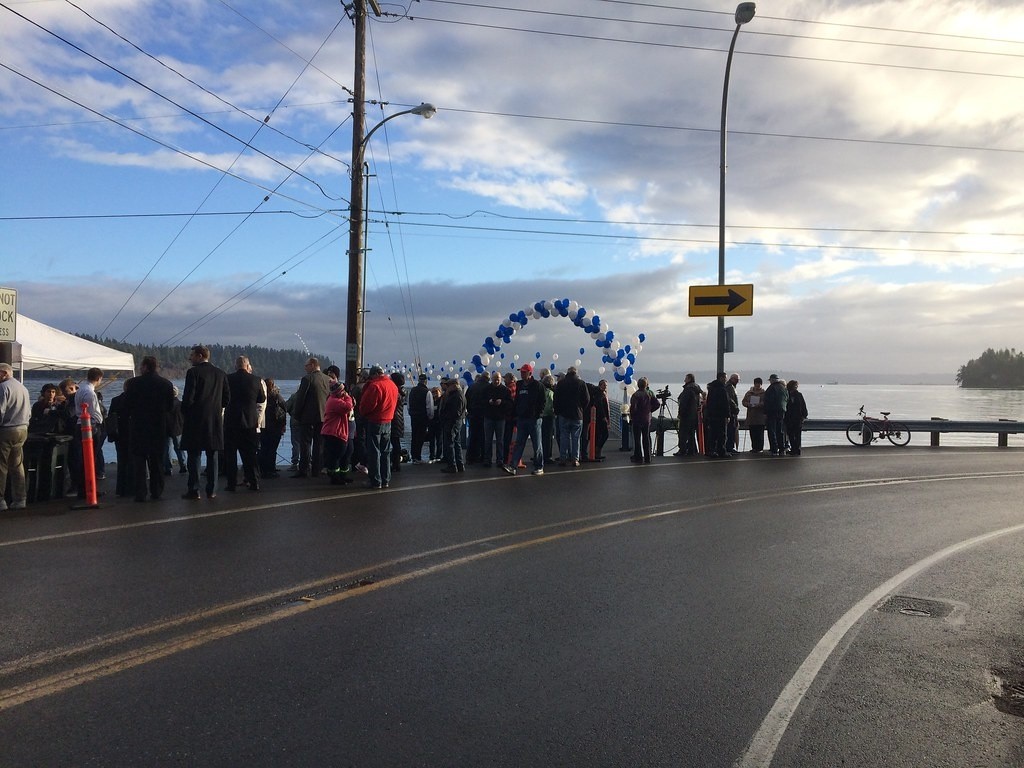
[846,404,911,448]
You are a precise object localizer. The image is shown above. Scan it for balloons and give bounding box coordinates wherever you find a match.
[371,296,646,391]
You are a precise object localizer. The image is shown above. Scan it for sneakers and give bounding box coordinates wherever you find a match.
[531,467,543,475]
[503,465,516,475]
[9,500,26,509]
[0,500,8,511]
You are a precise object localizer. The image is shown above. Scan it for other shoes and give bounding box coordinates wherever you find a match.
[557,461,580,467]
[673,446,801,458]
[224,460,505,492]
[121,466,217,503]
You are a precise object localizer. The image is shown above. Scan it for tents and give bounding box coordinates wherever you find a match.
[10,311,137,384]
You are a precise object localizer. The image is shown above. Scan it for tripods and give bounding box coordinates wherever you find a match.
[651,397,679,458]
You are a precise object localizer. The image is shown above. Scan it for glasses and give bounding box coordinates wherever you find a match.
[303,363,312,367]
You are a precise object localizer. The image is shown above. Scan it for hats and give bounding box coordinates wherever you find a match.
[501,373,514,379]
[785,380,798,390]
[357,370,369,378]
[517,364,532,371]
[327,365,340,378]
[419,374,427,380]
[768,374,779,381]
[554,372,565,377]
[461,371,471,378]
[328,380,345,395]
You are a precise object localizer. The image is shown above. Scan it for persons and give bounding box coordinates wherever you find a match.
[0,362,31,512]
[673,372,808,462]
[26,344,660,517]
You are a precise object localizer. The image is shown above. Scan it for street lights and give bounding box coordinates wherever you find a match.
[715,1,758,378]
[346,100,438,385]
